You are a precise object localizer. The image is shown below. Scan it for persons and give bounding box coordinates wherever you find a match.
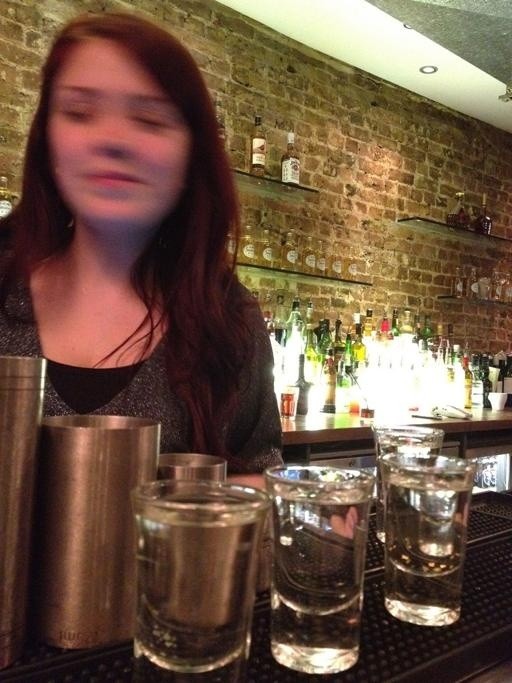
[0,9,285,474]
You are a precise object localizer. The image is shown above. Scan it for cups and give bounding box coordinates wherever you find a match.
[488,392,509,414]
[263,463,376,675]
[130,478,274,675]
[30,415,162,650]
[371,425,446,544]
[359,402,375,424]
[377,453,474,627]
[158,453,228,484]
[0,356,48,671]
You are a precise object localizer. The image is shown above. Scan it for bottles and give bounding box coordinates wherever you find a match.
[249,115,266,177]
[280,133,300,192]
[252,292,512,409]
[450,266,512,305]
[467,455,498,489]
[474,193,493,235]
[446,192,472,235]
[226,223,357,282]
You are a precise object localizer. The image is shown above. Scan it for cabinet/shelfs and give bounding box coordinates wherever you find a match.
[397,216,510,310]
[230,169,374,289]
